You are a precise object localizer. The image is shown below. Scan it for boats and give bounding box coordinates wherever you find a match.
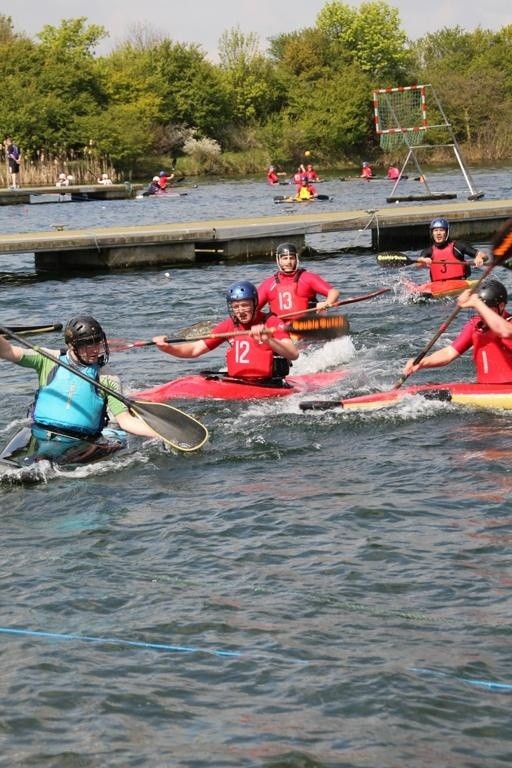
[137,173,426,203]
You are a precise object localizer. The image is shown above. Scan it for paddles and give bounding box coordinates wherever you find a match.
[274,195,329,200]
[387,219,512,389]
[1,325,209,451]
[378,251,509,268]
[105,328,277,352]
[277,288,390,319]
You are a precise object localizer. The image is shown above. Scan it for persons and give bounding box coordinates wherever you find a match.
[298,163,307,173]
[158,171,175,191]
[267,165,287,183]
[412,216,488,280]
[300,164,319,182]
[0,140,12,166]
[250,241,340,321]
[96,174,113,185]
[4,137,23,189]
[0,312,163,483]
[386,162,400,179]
[151,280,299,390]
[55,173,70,186]
[357,161,372,179]
[292,167,304,184]
[400,279,511,384]
[141,176,171,196]
[287,176,318,201]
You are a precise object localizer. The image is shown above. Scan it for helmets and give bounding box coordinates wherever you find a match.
[479,279,507,307]
[429,217,449,229]
[64,317,109,354]
[225,280,259,313]
[159,171,167,176]
[275,242,300,265]
[301,176,308,181]
[307,164,313,168]
[363,161,369,166]
[153,176,160,182]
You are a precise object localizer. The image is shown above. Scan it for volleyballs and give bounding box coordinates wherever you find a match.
[304,150,311,156]
